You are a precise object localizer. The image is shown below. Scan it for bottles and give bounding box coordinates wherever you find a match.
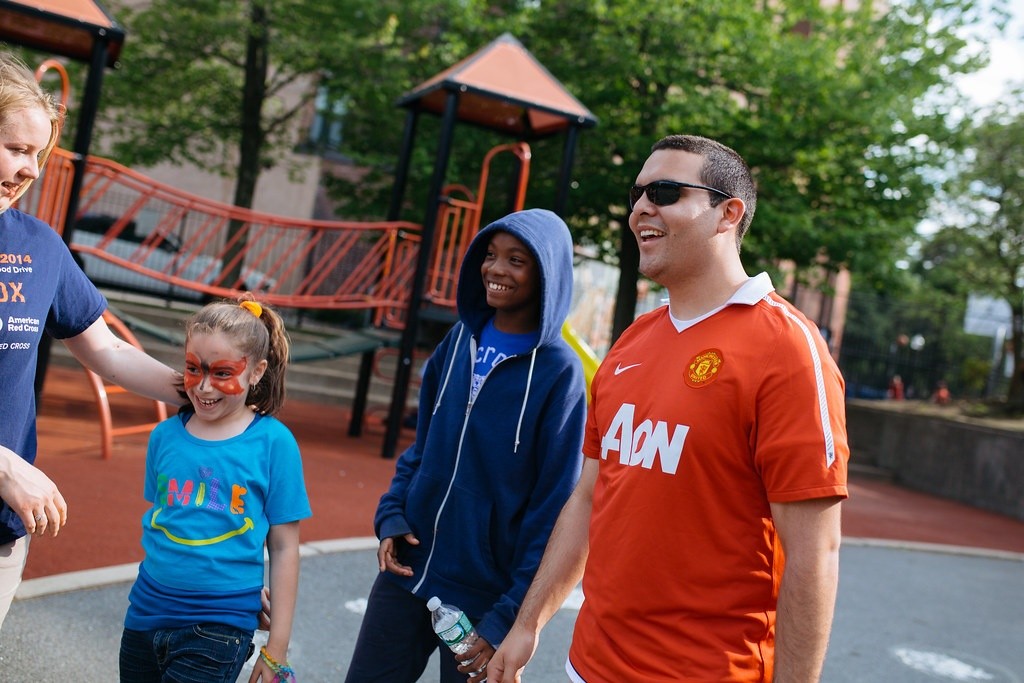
[427,596,488,682]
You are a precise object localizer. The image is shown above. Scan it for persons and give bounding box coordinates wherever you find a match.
[486,135,850,683]
[120,291,313,683]
[343,209,586,683]
[0,55,265,631]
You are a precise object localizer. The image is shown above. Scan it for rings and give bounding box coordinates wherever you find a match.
[35,512,46,521]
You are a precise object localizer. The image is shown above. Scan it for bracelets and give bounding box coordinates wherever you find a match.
[260,645,296,683]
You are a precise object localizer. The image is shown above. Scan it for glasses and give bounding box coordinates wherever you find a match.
[630,180,733,211]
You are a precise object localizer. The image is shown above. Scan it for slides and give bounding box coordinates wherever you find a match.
[562,323,601,403]
